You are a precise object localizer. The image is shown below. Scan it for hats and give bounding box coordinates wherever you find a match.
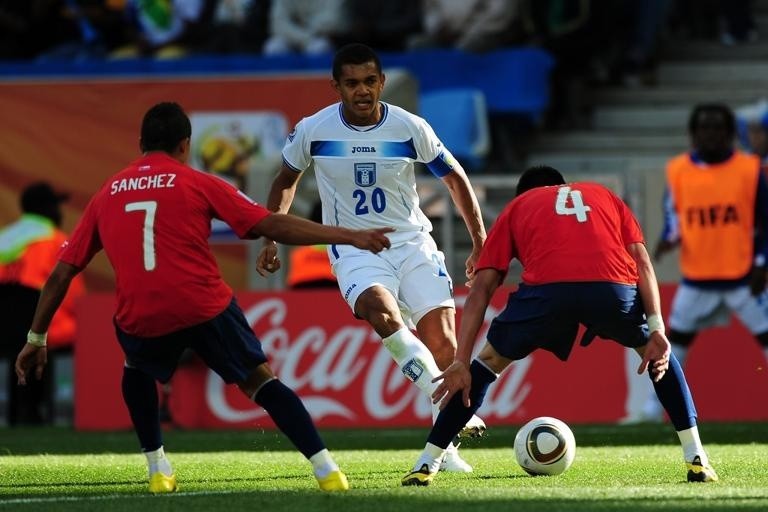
[18,181,67,212]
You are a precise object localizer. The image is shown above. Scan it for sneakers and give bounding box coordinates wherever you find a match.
[148,470,180,496]
[315,469,350,493]
[438,442,473,474]
[684,455,719,482]
[432,404,487,440]
[401,463,437,487]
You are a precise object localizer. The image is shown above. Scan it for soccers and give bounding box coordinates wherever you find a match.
[514,417,576,476]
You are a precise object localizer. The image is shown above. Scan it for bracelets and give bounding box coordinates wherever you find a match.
[644,314,666,335]
[25,329,48,348]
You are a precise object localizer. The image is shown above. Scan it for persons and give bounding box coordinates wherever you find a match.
[0,0,768,161]
[1,183,87,426]
[15,101,397,491]
[400,164,720,484]
[615,100,768,427]
[255,46,488,470]
